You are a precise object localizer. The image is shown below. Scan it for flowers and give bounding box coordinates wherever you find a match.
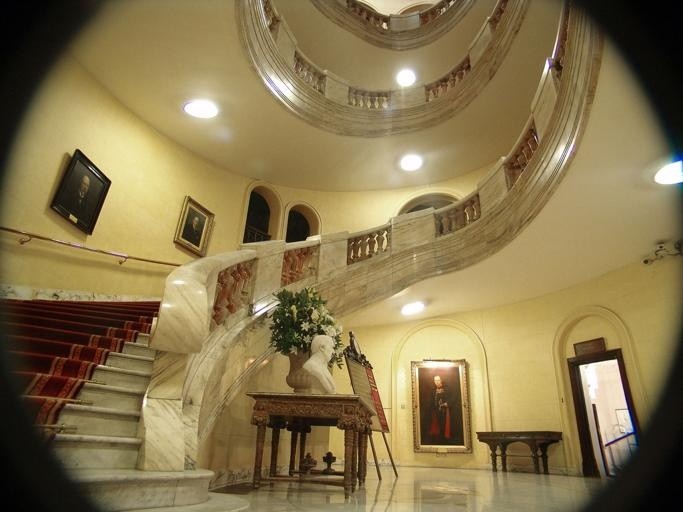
[268,284,345,376]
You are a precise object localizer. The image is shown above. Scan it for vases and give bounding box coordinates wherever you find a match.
[287,350,311,392]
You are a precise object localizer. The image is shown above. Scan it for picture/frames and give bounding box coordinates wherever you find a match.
[174,194,216,258]
[413,479,476,512]
[410,359,474,454]
[49,148,112,236]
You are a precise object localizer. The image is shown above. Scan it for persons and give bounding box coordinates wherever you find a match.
[302,334,337,394]
[424,373,462,444]
[182,216,201,247]
[60,175,95,226]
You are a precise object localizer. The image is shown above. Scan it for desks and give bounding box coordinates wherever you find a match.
[246,391,376,501]
[476,431,562,475]
